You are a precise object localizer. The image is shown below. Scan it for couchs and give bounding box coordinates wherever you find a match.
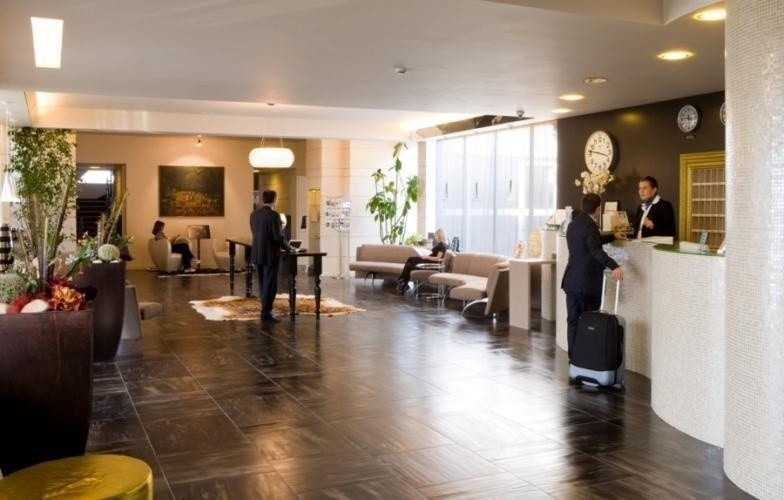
[426,251,509,320]
[147,237,192,274]
[212,236,245,271]
[347,243,450,298]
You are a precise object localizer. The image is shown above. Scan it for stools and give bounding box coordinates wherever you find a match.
[0,452,155,500]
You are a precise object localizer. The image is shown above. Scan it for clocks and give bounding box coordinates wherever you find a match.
[582,129,619,176]
[676,104,700,133]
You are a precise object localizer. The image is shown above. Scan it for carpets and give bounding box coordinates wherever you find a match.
[187,293,367,321]
[154,269,256,278]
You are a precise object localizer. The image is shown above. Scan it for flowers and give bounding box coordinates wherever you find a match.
[573,169,616,198]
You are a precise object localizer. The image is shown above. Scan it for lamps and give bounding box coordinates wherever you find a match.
[186,225,210,268]
[247,102,294,169]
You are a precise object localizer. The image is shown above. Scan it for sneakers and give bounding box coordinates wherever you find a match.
[191,259,202,267]
[184,267,196,273]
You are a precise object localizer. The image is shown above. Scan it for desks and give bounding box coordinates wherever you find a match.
[416,262,445,300]
[508,258,555,330]
[224,238,328,321]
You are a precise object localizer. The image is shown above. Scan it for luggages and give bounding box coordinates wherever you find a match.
[568,269,626,394]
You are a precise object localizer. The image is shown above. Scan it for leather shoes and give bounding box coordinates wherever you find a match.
[261,313,281,323]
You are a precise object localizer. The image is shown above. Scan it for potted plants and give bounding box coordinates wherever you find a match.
[0,126,94,478]
[70,185,138,364]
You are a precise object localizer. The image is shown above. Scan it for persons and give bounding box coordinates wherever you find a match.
[635,176,677,238]
[152,220,201,273]
[250,190,300,322]
[280,212,288,229]
[561,193,626,363]
[398,229,448,295]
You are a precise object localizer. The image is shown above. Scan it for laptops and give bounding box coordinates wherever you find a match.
[282,239,303,255]
